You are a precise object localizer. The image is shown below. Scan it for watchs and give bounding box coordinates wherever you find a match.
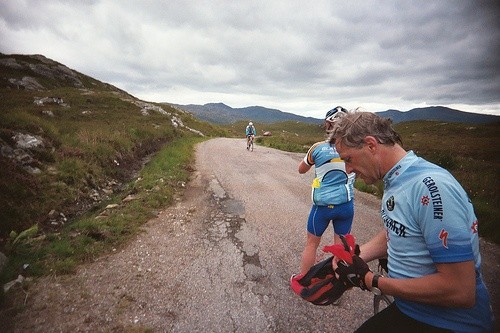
[372,274,384,296]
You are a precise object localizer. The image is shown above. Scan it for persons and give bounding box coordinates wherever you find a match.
[327,110,496,333]
[245,122,256,150]
[290,106,358,282]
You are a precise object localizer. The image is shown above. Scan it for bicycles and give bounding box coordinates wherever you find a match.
[246,134,255,152]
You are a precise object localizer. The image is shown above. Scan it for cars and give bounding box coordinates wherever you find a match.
[263,131,273,136]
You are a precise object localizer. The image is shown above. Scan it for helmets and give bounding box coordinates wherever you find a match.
[325,106,349,125]
